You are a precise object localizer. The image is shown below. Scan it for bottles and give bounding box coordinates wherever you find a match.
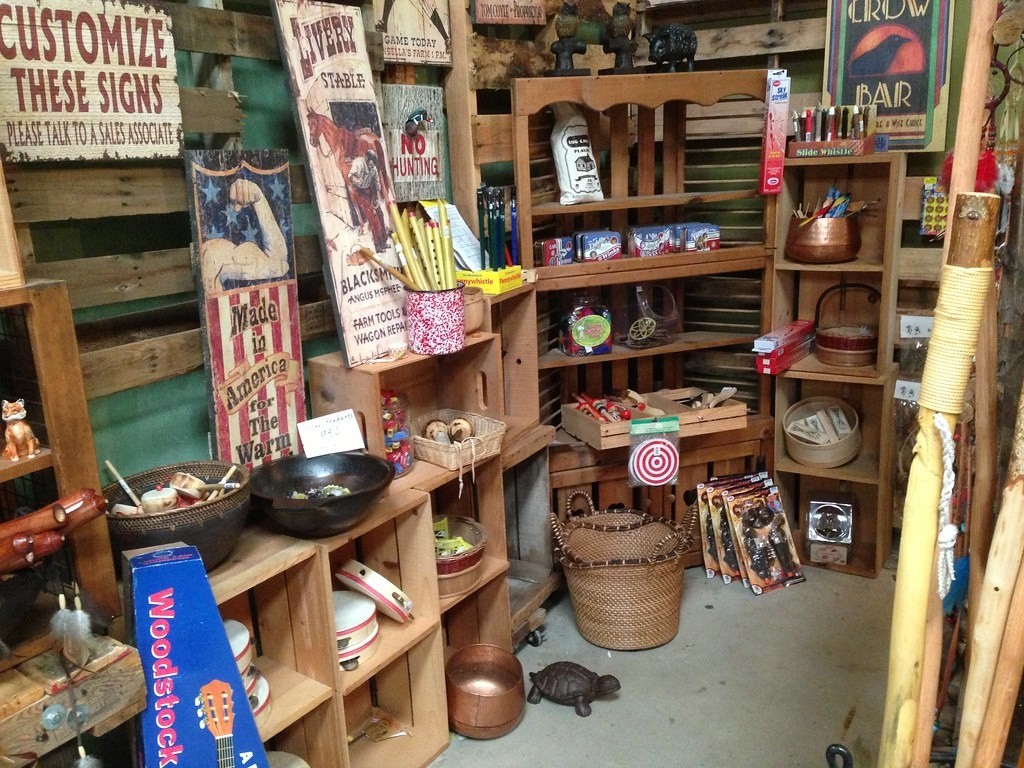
[557,294,612,359]
[380,388,415,480]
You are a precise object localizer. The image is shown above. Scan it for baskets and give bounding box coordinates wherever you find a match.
[433,514,487,575]
[407,408,507,470]
[101,462,250,531]
[815,283,881,351]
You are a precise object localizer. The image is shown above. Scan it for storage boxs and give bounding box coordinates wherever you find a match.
[789,129,876,158]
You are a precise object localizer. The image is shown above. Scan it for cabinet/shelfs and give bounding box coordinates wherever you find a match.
[0,67,907,768]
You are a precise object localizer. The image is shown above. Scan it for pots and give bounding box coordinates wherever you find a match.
[247,450,397,540]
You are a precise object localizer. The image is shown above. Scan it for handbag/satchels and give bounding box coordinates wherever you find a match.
[551,490,698,650]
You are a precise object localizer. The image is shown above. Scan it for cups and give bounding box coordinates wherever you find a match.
[403,280,467,356]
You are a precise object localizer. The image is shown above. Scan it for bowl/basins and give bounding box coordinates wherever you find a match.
[782,395,862,470]
[444,643,527,741]
[99,460,250,576]
[787,211,862,264]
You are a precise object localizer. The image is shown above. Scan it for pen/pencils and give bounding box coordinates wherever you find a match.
[792,100,871,142]
[793,192,852,231]
[360,198,458,291]
[476,182,517,271]
